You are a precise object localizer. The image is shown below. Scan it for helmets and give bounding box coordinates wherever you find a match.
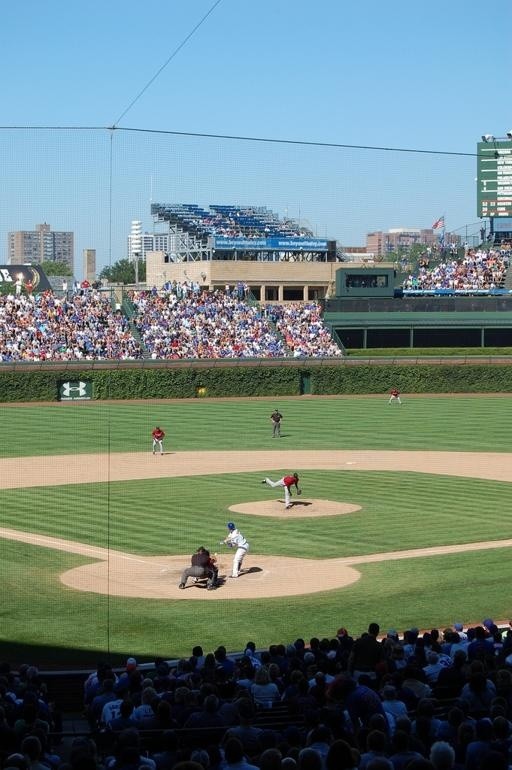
[228,522,235,529]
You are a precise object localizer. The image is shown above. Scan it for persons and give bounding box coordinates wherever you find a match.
[261,472,301,509]
[198,211,306,238]
[401,239,511,298]
[388,387,402,405]
[219,523,249,578]
[179,546,218,590]
[151,425,165,456]
[1,619,512,770]
[270,409,283,438]
[1,273,343,363]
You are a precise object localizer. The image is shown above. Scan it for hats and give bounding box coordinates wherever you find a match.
[127,658,136,665]
[294,473,298,477]
[198,547,205,553]
[336,628,347,638]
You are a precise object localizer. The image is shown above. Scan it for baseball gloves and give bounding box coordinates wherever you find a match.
[211,555,217,563]
[296,490,301,495]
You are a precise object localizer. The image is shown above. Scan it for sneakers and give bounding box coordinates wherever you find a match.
[207,583,216,590]
[179,584,185,588]
[287,505,292,508]
[262,480,267,483]
[229,576,237,579]
